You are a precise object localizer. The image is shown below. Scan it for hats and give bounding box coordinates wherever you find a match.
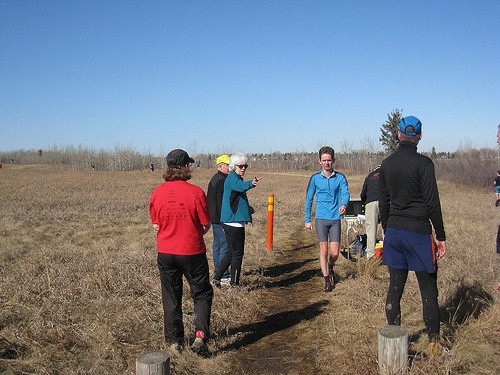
[166,148,194,168]
[216,155,231,165]
[399,115,422,136]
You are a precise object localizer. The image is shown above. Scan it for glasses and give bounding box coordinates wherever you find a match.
[236,165,248,168]
[189,163,193,166]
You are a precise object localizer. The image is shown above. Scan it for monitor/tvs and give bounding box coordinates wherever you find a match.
[344,199,365,218]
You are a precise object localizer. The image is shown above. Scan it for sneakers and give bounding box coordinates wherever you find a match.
[325,280,331,292]
[170,343,183,353]
[211,268,242,290]
[191,339,215,358]
[328,262,335,274]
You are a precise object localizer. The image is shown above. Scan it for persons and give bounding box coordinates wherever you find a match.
[378,115,451,351]
[209,152,262,292]
[304,146,350,291]
[494,170,500,206]
[206,154,231,295]
[497,124,500,147]
[149,149,214,358]
[360,164,385,261]
